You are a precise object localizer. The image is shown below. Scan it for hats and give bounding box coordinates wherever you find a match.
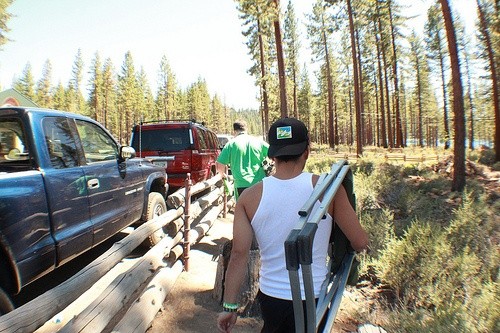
[268,117,309,158]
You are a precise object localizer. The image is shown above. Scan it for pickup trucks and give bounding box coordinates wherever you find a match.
[0,106,168,311]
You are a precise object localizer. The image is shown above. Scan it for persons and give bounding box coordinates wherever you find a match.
[216,121,271,200]
[217,118,369,333]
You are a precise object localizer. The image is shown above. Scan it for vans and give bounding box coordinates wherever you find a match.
[126,119,222,193]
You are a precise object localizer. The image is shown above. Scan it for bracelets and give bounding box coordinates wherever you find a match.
[223,303,237,312]
